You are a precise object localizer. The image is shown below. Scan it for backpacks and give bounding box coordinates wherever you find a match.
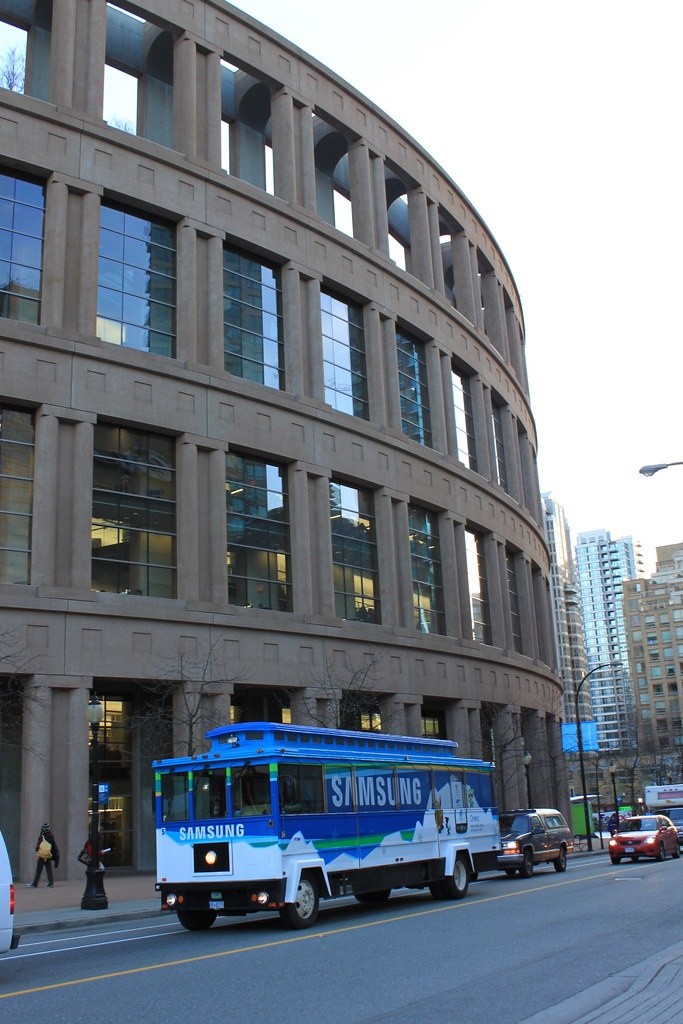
[37,835,52,862]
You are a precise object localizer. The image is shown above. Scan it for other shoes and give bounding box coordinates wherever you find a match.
[45,883,53,888]
[26,883,37,888]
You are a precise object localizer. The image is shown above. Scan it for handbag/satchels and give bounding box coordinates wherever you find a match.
[78,842,92,865]
[98,861,105,870]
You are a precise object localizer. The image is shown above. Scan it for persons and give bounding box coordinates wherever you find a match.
[85,838,105,869]
[26,823,56,888]
[608,816,617,838]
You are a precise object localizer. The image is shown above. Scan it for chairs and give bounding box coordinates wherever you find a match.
[279,774,301,814]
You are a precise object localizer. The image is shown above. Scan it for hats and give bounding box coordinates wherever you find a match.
[41,823,50,833]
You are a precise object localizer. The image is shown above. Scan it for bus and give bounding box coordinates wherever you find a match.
[151,721,505,931]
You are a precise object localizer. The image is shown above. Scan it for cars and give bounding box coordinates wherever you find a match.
[609,814,681,864]
[591,784,683,849]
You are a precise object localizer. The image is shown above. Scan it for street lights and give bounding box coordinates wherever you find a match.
[521,750,534,808]
[609,760,621,831]
[79,688,110,910]
[574,662,623,851]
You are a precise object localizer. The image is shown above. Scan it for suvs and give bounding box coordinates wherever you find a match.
[493,808,573,878]
